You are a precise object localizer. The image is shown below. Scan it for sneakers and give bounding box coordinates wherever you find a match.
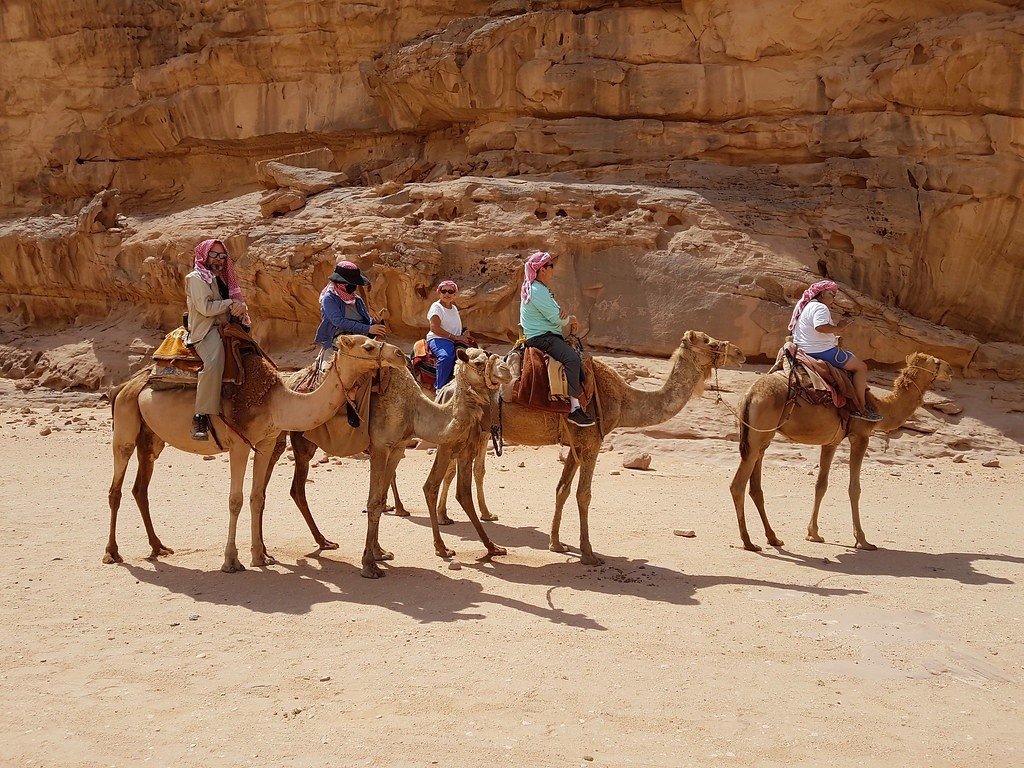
[191,416,209,441]
[568,407,595,427]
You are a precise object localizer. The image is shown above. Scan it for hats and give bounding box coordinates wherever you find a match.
[328,261,369,284]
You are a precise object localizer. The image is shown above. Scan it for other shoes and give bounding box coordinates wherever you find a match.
[346,400,360,428]
[849,407,883,422]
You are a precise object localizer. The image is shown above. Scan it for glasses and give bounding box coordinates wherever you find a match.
[208,250,228,259]
[440,289,455,295]
[544,263,554,268]
[826,290,836,299]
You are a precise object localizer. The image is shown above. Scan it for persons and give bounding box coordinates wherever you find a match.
[788,280,883,421]
[312,260,386,387]
[427,280,469,396]
[518,252,595,427]
[184,239,251,440]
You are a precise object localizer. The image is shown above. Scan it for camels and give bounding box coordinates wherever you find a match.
[100,333,407,574]
[410,329,747,567]
[730,351,955,557]
[261,344,514,582]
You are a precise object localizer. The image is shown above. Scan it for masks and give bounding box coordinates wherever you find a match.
[344,283,357,294]
